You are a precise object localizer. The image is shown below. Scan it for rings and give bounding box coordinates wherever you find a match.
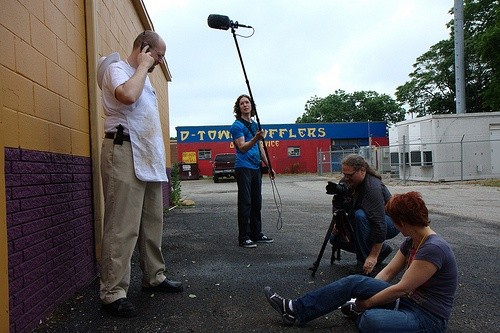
[369,266,371,268]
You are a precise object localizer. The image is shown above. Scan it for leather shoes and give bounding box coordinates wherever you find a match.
[144,277,183,292]
[101,297,138,318]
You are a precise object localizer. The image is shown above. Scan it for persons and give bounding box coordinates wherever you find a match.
[97,30,183,317]
[329,153,400,276]
[264,191,459,333]
[230,94,276,248]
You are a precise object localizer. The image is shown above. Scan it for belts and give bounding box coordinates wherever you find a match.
[105,132,129,141]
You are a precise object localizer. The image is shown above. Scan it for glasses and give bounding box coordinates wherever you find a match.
[340,170,357,178]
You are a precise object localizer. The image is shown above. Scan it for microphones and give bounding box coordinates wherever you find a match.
[208,14,252,31]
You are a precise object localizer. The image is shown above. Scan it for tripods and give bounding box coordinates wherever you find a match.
[310,195,366,278]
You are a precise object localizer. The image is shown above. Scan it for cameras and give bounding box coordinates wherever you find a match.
[325,181,348,196]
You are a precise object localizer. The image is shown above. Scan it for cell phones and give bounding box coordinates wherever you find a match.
[141,42,149,54]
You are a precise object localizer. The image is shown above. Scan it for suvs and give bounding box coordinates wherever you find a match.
[210,153,237,183]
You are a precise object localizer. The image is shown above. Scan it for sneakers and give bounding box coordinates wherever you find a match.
[340,301,363,319]
[240,238,257,248]
[264,286,297,324]
[257,235,274,243]
[348,263,370,274]
[375,244,393,266]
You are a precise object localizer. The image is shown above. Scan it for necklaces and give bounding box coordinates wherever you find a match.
[403,226,430,271]
